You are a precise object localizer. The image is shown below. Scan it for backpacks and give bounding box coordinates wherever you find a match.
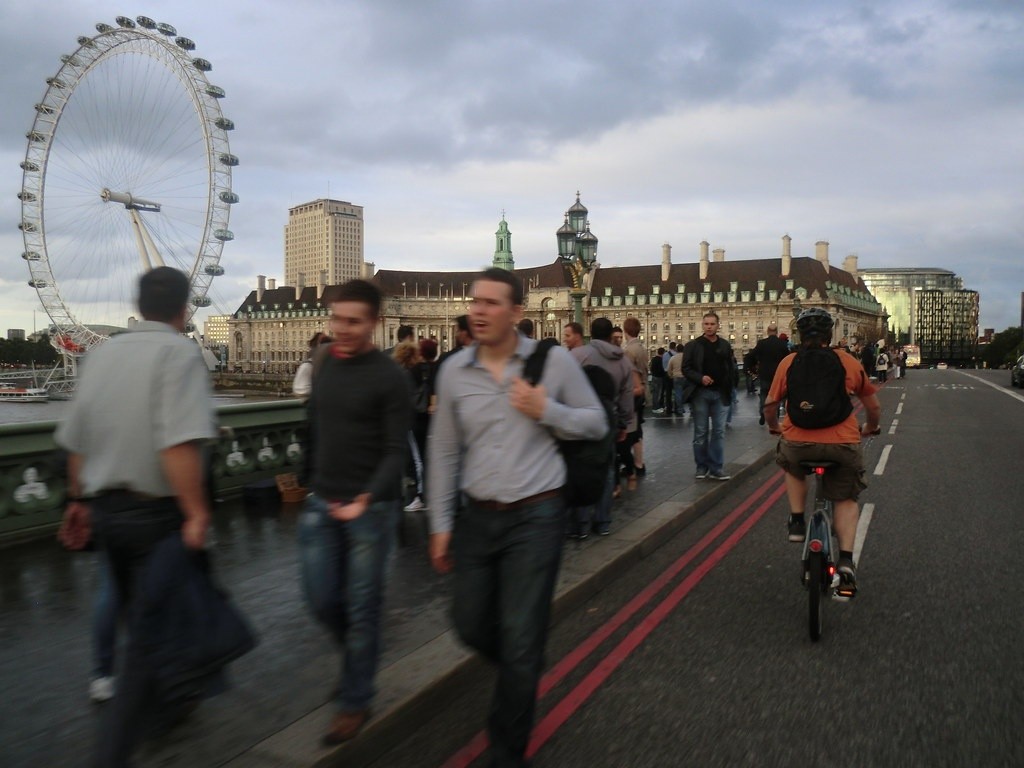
[786,343,854,429]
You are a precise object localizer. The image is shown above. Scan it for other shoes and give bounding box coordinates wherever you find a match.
[612,489,622,498]
[468,743,524,768]
[563,526,589,539]
[634,463,646,479]
[321,708,368,745]
[591,526,609,536]
[628,477,637,490]
[90,678,116,702]
[620,466,633,478]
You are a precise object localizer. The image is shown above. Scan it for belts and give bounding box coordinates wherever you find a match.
[464,488,561,512]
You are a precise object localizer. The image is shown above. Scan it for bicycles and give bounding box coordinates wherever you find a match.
[768,426,882,643]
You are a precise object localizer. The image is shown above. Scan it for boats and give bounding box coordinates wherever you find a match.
[0,382,50,402]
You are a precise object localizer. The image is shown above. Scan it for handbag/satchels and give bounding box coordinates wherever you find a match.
[525,337,620,507]
[141,548,261,697]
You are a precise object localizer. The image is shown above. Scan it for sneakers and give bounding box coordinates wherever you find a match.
[836,560,858,597]
[402,496,429,512]
[695,468,710,478]
[709,469,731,480]
[788,515,806,542]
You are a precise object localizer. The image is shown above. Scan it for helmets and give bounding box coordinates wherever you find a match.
[796,307,834,329]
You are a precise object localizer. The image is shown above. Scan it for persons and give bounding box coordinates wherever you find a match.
[427,267,610,768]
[299,279,411,746]
[292,314,648,539]
[649,342,740,426]
[763,307,881,590]
[743,322,907,425]
[53,266,217,702]
[681,313,733,480]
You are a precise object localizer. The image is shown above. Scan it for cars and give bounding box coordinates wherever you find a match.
[1008,355,1024,388]
[936,362,947,370]
